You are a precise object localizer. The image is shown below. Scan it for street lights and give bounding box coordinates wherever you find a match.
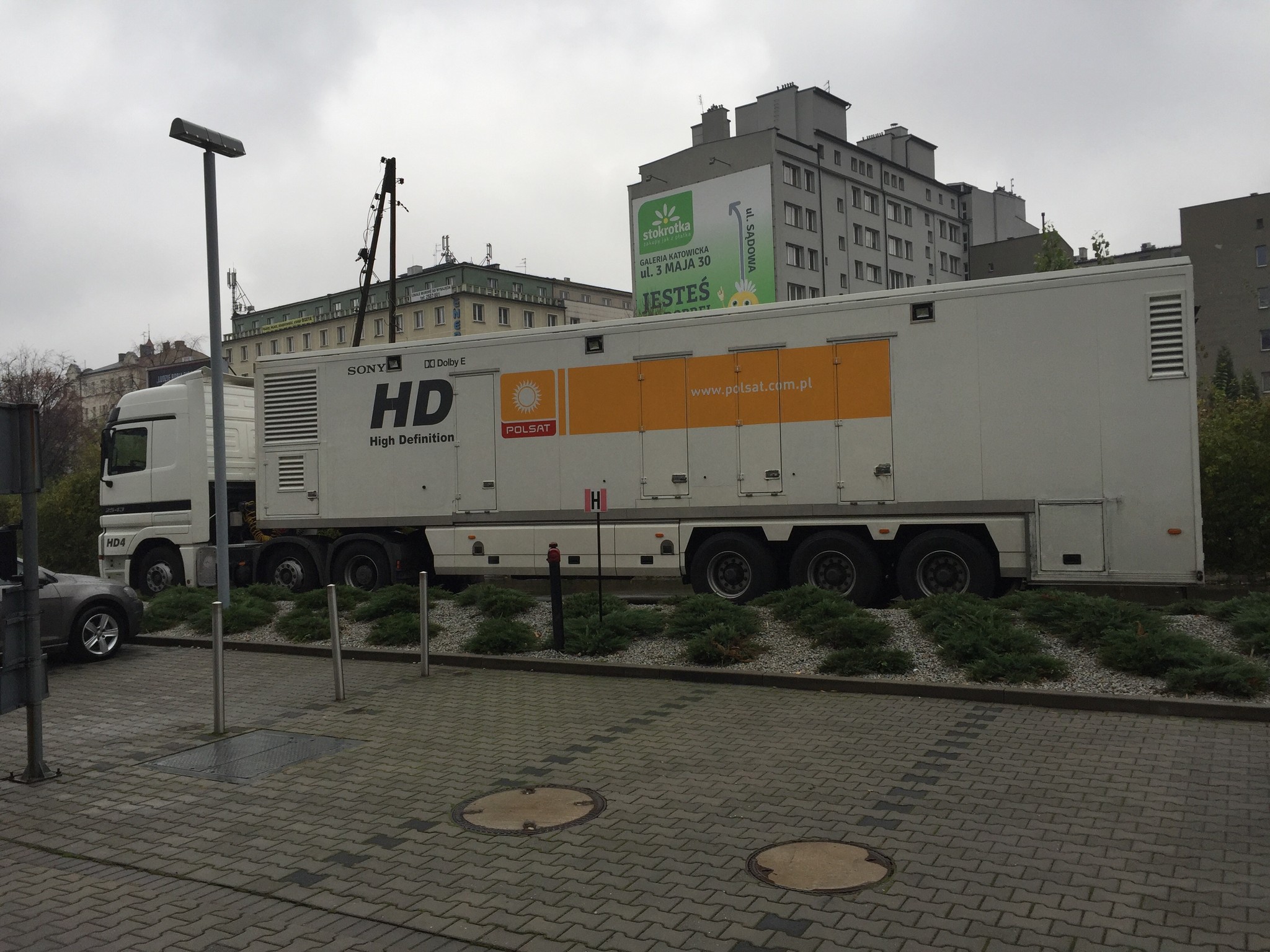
[167,115,248,611]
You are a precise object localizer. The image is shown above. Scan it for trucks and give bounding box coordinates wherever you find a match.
[95,254,1207,599]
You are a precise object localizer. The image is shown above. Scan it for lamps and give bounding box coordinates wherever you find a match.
[646,175,667,183]
[709,156,731,166]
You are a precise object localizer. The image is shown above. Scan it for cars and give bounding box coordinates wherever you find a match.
[0,557,145,674]
